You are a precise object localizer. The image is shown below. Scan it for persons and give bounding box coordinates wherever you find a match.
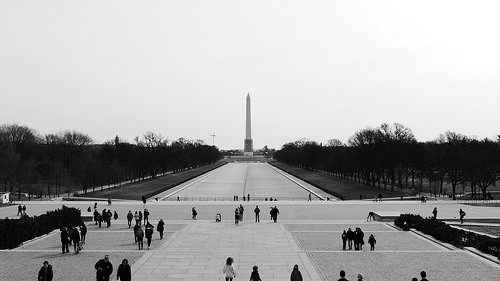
[290,265,303,281]
[61,222,88,254]
[308,192,312,202]
[192,207,198,220]
[412,271,429,281]
[157,219,165,240]
[340,194,383,202]
[17,204,26,216]
[95,255,113,281]
[37,261,54,281]
[127,209,154,250]
[216,194,279,225]
[358,273,364,281]
[368,234,376,251]
[88,198,118,229]
[337,270,349,281]
[460,209,465,224]
[222,257,236,281]
[117,259,131,281]
[341,226,365,250]
[250,266,262,281]
[141,195,146,206]
[432,207,438,219]
[367,212,375,221]
